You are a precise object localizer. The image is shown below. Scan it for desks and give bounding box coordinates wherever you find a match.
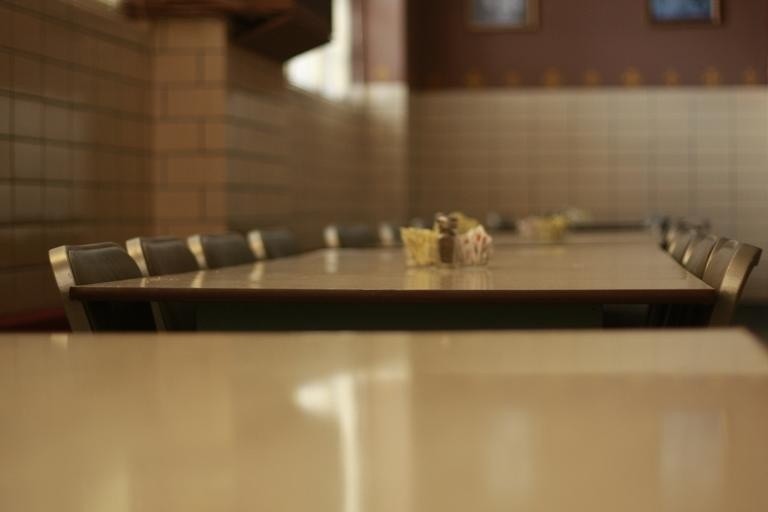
[72,229,716,329]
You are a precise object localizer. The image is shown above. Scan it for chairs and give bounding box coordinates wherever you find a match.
[189,232,255,275]
[127,235,199,278]
[247,216,379,268]
[45,240,142,330]
[665,221,764,331]
[378,214,640,248]
